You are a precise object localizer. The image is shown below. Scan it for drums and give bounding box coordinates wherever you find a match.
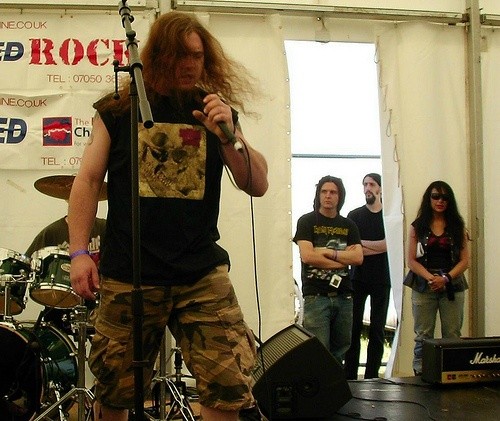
[28,245,85,309]
[0,245,33,315]
[0,318,80,421]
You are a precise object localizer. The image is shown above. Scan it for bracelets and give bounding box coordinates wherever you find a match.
[69,250,92,258]
[333,248,337,260]
[221,126,235,145]
[443,273,451,282]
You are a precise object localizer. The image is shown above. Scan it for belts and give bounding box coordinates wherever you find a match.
[318,292,340,297]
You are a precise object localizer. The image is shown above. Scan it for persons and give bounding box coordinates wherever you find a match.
[403,180,473,377]
[293,175,364,376]
[347,173,391,379]
[68,13,269,421]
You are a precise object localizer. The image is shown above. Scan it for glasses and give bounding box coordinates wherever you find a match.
[431,193,449,201]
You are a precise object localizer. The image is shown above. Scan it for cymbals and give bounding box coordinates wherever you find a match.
[34,175,111,201]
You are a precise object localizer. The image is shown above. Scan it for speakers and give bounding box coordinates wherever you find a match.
[247,324,354,421]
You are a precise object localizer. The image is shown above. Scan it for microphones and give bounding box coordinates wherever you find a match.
[216,121,244,153]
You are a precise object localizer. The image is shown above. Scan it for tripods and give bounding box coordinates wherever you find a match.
[37,294,195,421]
